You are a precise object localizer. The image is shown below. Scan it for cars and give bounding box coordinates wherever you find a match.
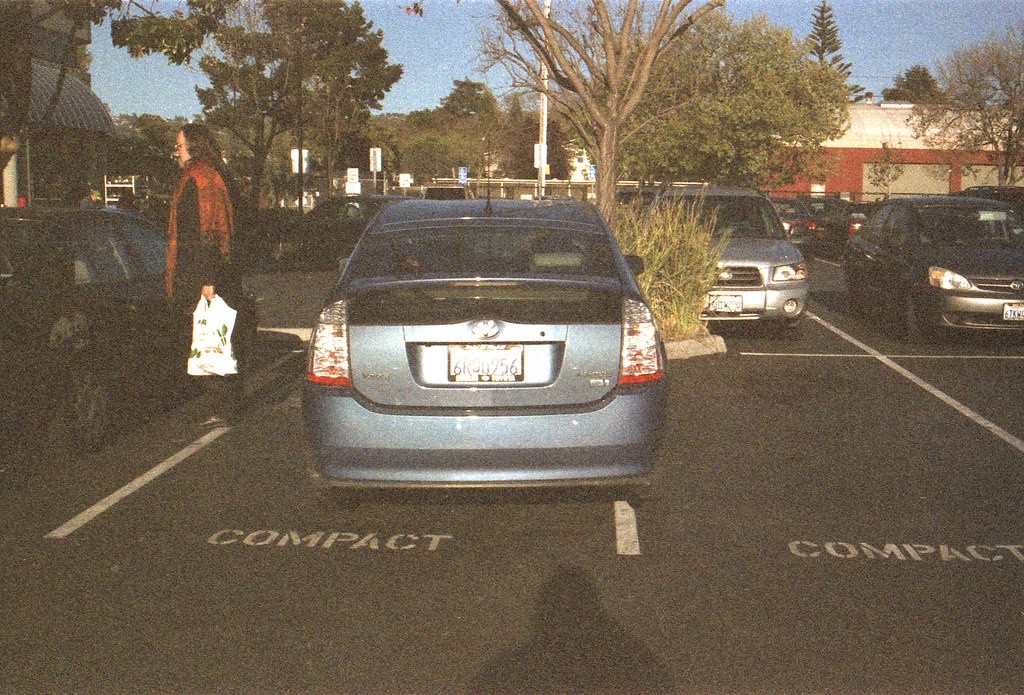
[760,184,880,260]
[303,195,669,489]
[271,196,395,274]
[1,205,257,458]
[843,195,1024,345]
[422,184,472,200]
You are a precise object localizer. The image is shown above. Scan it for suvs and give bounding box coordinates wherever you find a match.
[952,185,1024,208]
[639,186,809,339]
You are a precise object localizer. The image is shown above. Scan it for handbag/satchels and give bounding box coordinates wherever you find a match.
[187,294,238,376]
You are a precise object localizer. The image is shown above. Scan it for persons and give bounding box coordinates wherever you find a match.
[80,191,103,209]
[164,124,247,428]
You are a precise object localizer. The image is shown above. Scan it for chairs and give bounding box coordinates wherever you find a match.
[528,252,588,275]
[952,219,980,239]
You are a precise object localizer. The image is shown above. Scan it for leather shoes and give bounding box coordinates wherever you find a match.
[197,414,236,430]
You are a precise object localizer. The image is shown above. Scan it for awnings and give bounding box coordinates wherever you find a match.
[24,62,116,134]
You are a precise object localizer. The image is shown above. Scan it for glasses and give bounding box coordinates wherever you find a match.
[174,142,188,151]
